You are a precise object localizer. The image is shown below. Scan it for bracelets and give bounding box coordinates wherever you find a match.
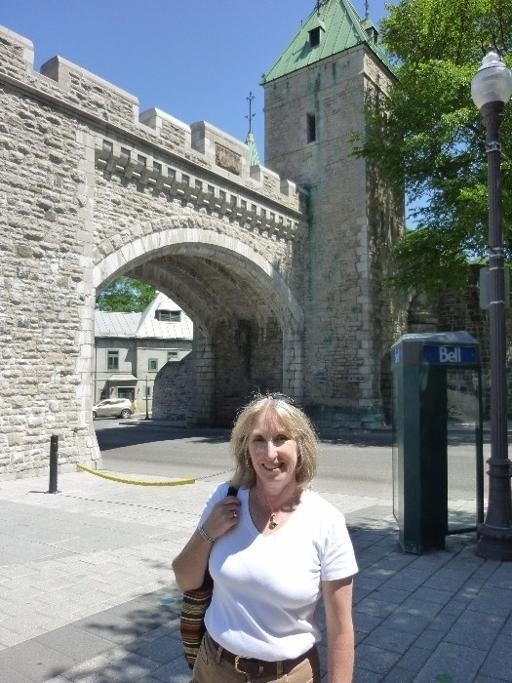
[197,524,216,544]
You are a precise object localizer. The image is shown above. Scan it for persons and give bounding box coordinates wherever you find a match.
[172,390,359,683]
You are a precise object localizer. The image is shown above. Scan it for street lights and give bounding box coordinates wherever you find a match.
[471,52,512,561]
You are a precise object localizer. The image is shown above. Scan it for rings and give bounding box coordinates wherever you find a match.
[233,511,238,518]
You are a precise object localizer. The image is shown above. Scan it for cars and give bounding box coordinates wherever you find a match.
[93,399,134,420]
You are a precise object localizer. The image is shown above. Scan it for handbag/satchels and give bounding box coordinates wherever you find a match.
[179,570,214,671]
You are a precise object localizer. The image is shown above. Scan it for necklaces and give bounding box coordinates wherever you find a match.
[269,511,278,528]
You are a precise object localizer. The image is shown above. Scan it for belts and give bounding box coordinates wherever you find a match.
[208,637,309,680]
